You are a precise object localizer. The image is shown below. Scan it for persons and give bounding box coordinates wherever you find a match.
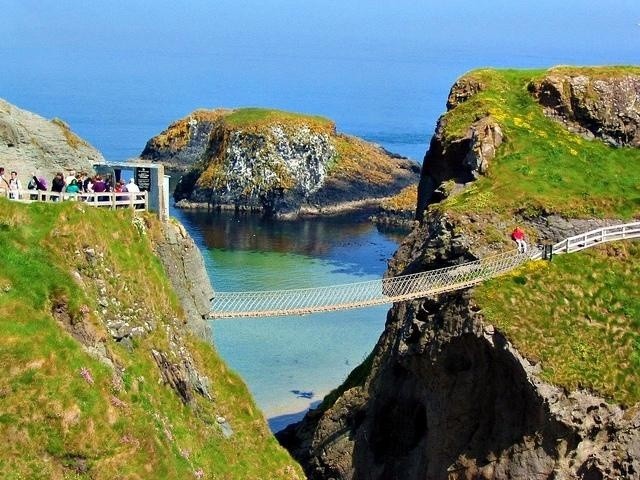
[511,226,526,253]
[7,170,25,201]
[28,171,144,209]
[0,166,7,194]
